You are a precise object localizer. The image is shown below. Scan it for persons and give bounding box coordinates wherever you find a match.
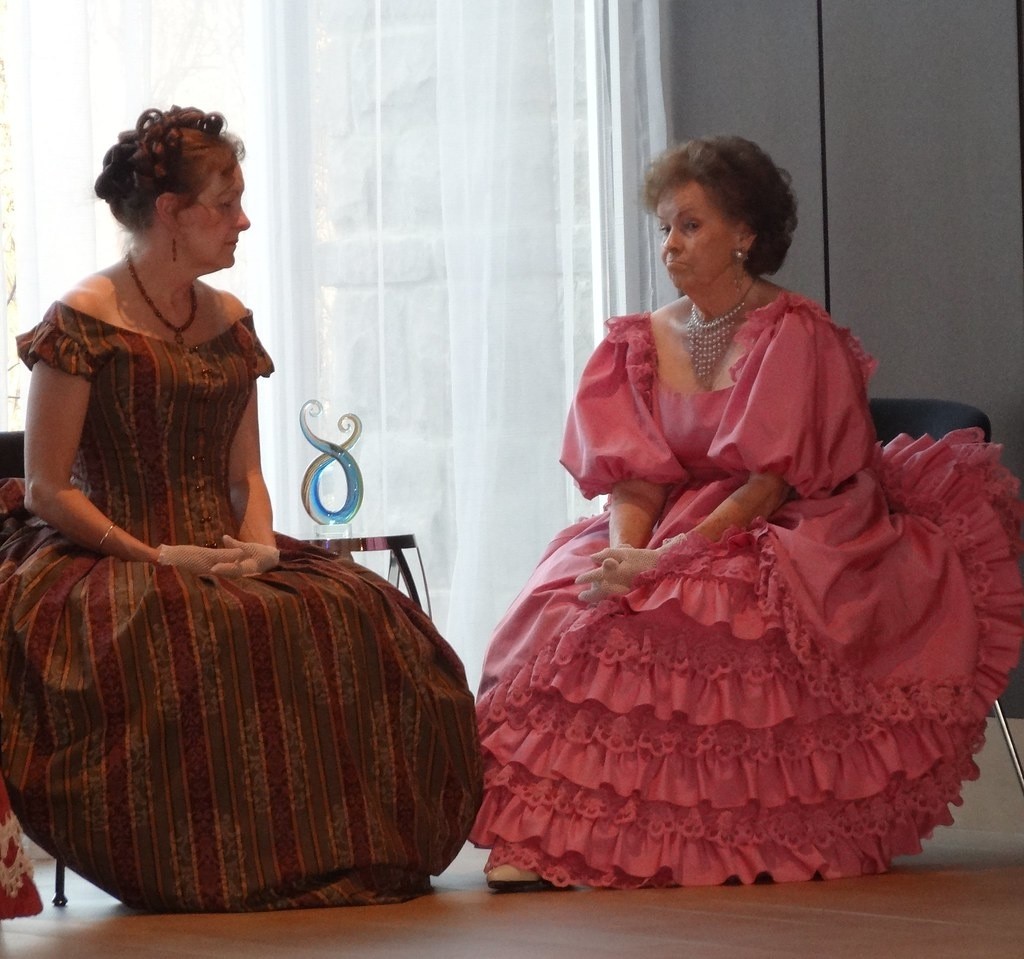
[469,134,1024,888]
[2,108,481,911]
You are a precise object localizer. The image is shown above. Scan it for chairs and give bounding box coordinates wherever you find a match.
[868,396,1022,798]
[0,432,78,906]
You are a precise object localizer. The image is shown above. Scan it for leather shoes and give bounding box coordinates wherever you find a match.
[487,866,538,889]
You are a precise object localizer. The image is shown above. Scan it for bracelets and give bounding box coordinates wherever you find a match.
[99,524,115,545]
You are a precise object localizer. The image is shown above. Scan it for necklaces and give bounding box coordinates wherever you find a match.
[688,278,757,387]
[126,251,197,344]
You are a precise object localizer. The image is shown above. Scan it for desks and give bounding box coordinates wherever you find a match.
[294,527,436,627]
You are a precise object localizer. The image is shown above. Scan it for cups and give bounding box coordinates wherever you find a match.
[313,523,351,563]
